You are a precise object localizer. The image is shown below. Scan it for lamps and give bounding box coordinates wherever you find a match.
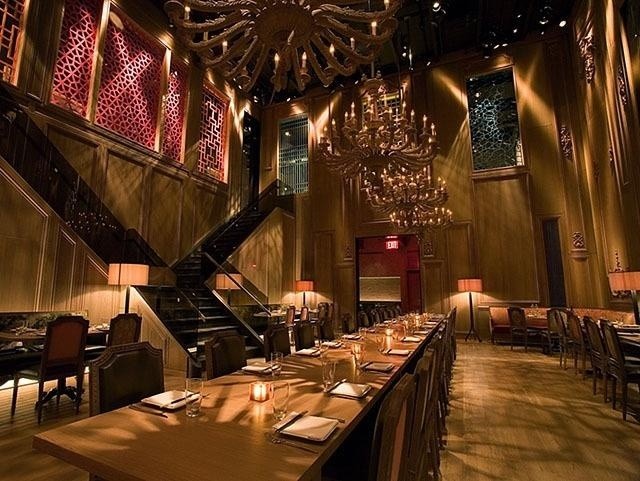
[610,270,640,330]
[457,277,487,345]
[107,263,150,313]
[294,280,315,317]
[217,272,241,309]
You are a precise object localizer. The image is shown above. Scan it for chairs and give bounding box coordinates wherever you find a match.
[504,304,639,420]
[366,306,456,480]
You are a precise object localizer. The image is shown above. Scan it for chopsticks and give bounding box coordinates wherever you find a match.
[170,390,199,405]
[328,379,344,391]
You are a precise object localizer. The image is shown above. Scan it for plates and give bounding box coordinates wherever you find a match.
[140,389,200,413]
[327,381,372,400]
[272,409,339,444]
[241,362,281,376]
[295,315,441,374]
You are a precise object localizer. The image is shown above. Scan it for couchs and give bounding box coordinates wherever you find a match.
[488,304,637,343]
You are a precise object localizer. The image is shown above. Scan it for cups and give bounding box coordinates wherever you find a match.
[270,352,283,378]
[185,378,203,417]
[270,382,292,420]
[321,360,335,390]
[314,310,431,369]
